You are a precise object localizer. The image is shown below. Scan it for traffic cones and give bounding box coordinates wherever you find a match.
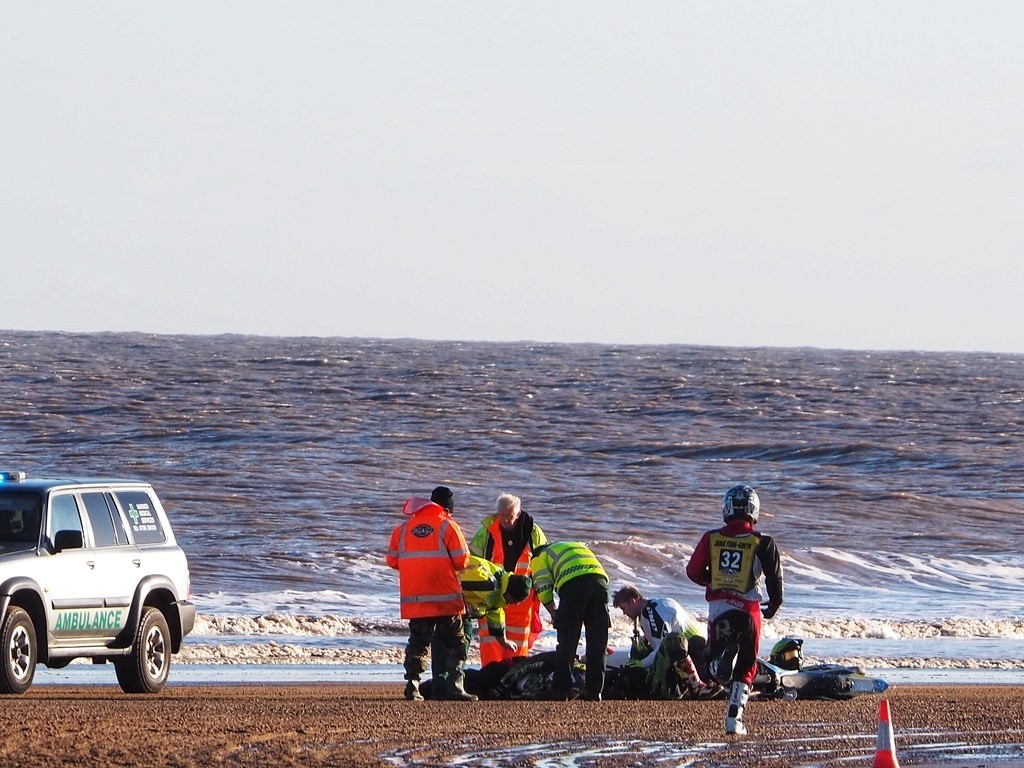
[871,699,901,768]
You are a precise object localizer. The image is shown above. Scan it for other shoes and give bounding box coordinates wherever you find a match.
[430,679,450,700]
[582,688,601,700]
[550,689,571,699]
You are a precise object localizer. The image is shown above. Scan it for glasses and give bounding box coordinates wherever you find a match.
[499,511,517,520]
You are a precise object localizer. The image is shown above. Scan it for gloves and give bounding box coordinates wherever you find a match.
[761,600,779,619]
[626,660,644,668]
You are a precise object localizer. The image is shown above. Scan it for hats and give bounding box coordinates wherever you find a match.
[431,486,455,508]
[509,575,532,601]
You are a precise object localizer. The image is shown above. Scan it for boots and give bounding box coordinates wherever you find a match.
[403,679,425,701]
[446,659,479,702]
[673,655,724,699]
[725,681,751,735]
[709,643,740,685]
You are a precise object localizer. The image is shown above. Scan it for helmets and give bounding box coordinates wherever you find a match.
[770,638,803,670]
[722,485,761,524]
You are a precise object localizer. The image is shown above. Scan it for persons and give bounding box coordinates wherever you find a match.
[529,541,612,701]
[386,486,724,701]
[468,491,549,669]
[685,485,784,735]
[386,486,479,701]
[431,555,533,701]
[612,584,706,669]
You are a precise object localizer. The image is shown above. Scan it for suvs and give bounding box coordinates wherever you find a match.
[0,471,195,695]
